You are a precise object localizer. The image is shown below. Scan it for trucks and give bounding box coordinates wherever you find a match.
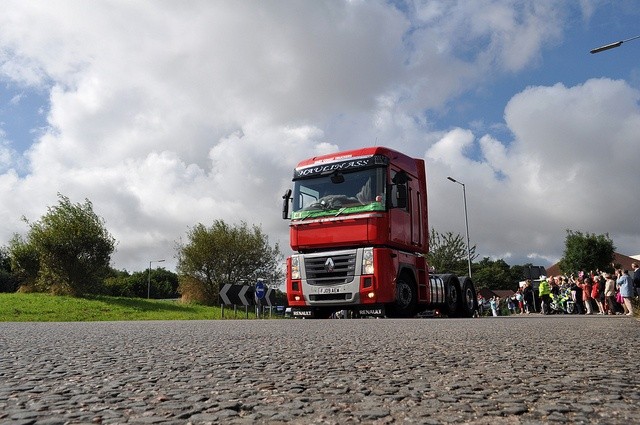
[282,146,477,319]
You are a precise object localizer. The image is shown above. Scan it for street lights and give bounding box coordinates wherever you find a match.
[147,260,165,300]
[447,177,472,278]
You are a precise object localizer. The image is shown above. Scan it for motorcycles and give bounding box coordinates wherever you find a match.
[549,288,575,314]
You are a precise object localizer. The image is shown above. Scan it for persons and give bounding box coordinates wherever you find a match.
[631,261,640,310]
[472,263,634,316]
